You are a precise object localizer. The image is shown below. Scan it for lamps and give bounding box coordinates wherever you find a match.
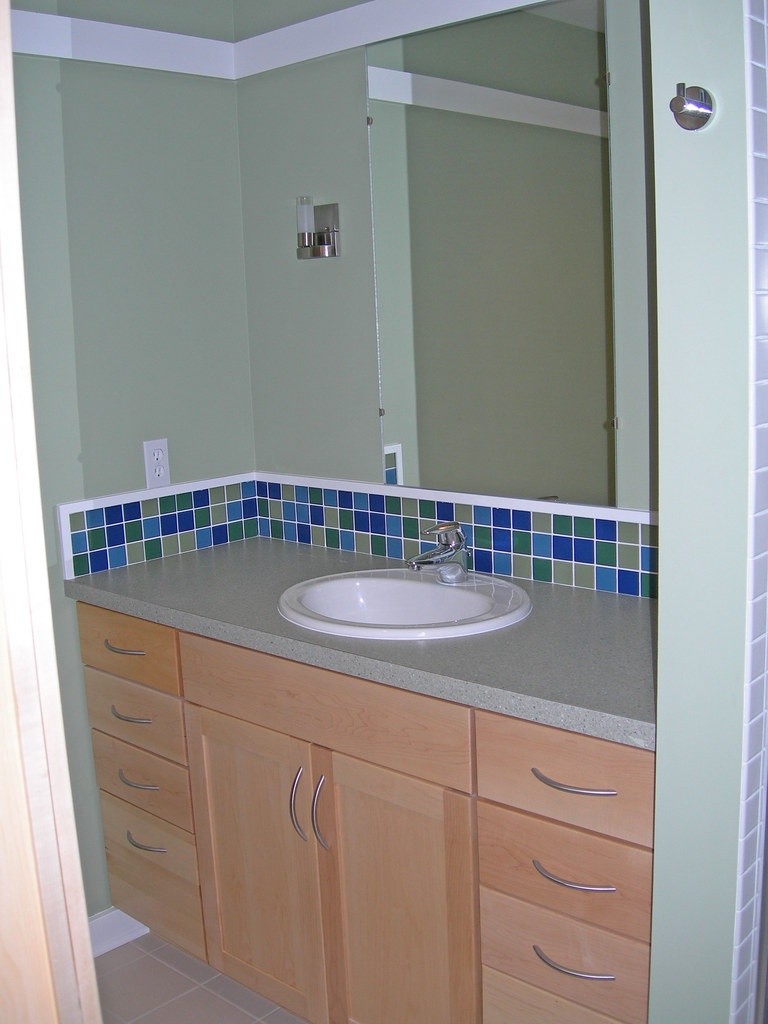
[295,197,342,260]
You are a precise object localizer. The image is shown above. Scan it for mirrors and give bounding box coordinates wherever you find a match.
[362,0,616,507]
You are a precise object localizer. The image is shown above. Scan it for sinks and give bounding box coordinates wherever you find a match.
[277,561,536,641]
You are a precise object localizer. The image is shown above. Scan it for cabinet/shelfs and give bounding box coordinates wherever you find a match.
[76,601,656,1024]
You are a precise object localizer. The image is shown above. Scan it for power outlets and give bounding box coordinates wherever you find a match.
[143,438,170,489]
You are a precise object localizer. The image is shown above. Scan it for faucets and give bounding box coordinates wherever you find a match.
[404,519,472,584]
